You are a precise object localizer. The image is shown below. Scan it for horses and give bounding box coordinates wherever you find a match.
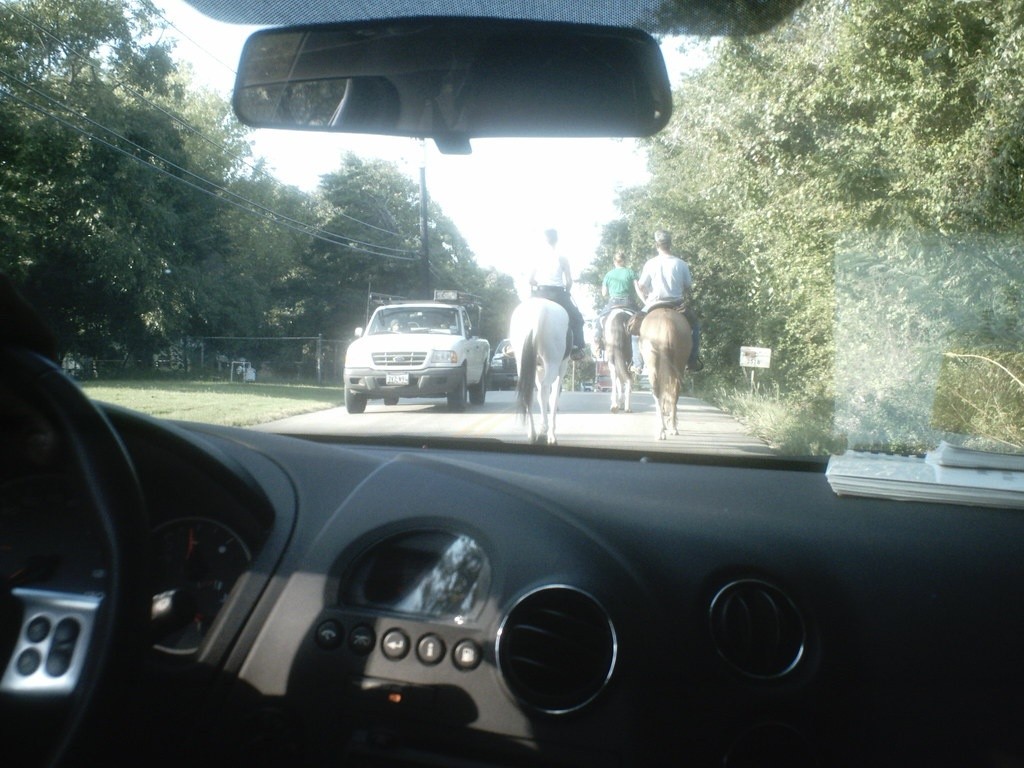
[602,308,693,439]
[509,298,573,446]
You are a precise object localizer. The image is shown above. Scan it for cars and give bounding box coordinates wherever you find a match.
[489,338,518,391]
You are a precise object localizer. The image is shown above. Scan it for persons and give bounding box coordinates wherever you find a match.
[528,229,586,359]
[595,252,638,350]
[638,230,703,372]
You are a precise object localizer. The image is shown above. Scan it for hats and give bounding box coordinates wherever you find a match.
[654,230,672,245]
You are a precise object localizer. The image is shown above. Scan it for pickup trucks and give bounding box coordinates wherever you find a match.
[341,301,492,414]
